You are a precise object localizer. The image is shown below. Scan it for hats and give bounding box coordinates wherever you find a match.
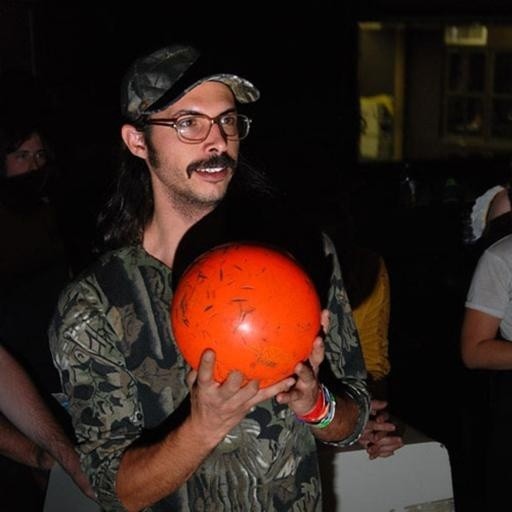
[120,42,260,124]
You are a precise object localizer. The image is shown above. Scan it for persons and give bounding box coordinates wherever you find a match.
[50,45,404,511]
[456,178,512,372]
[2,119,99,511]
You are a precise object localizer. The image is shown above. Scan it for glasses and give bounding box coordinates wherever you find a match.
[145,113,253,146]
